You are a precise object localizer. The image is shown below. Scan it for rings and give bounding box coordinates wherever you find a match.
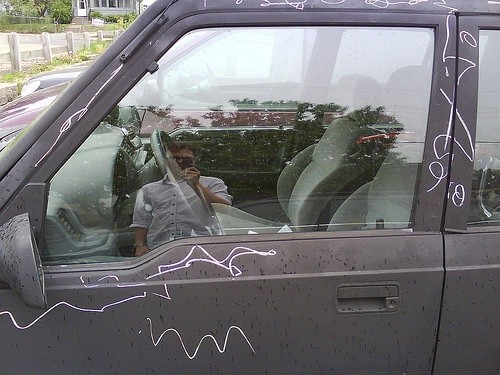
[196,170,199,172]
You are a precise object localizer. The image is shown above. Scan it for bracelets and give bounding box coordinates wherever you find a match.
[133,241,147,248]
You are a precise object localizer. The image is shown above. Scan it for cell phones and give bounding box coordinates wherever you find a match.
[170,156,193,171]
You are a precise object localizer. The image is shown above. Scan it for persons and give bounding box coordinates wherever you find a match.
[129,141,234,258]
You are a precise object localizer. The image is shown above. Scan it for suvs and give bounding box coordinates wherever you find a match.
[1,0,499,375]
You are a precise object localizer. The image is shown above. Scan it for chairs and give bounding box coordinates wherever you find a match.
[325,65,438,234]
[202,72,387,240]
